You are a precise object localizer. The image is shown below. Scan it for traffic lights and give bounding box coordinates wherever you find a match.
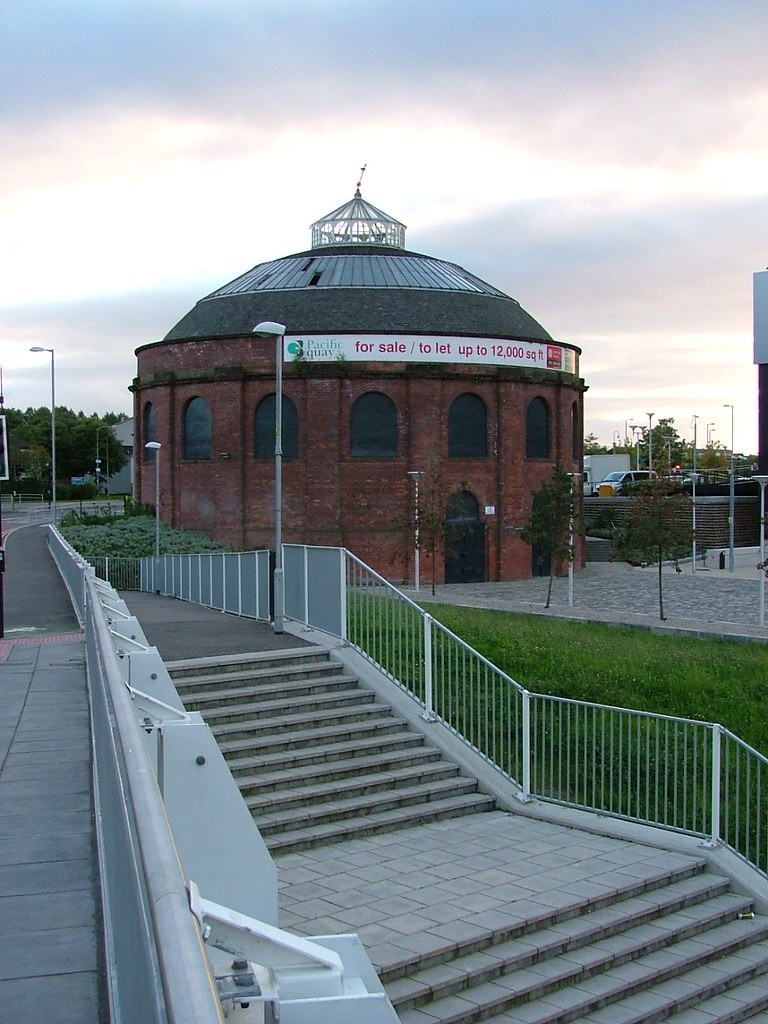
[0,415,9,480]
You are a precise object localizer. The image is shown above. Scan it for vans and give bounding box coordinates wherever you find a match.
[594,471,658,497]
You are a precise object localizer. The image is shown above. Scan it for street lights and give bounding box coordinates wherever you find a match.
[252,322,286,635]
[567,472,582,607]
[723,405,733,468]
[629,426,647,471]
[663,437,676,480]
[96,425,118,498]
[408,471,426,591]
[647,413,655,480]
[751,475,768,627]
[625,419,633,439]
[687,473,701,574]
[707,423,715,442]
[145,441,162,556]
[29,347,57,524]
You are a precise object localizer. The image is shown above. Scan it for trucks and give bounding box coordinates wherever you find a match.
[583,454,630,497]
